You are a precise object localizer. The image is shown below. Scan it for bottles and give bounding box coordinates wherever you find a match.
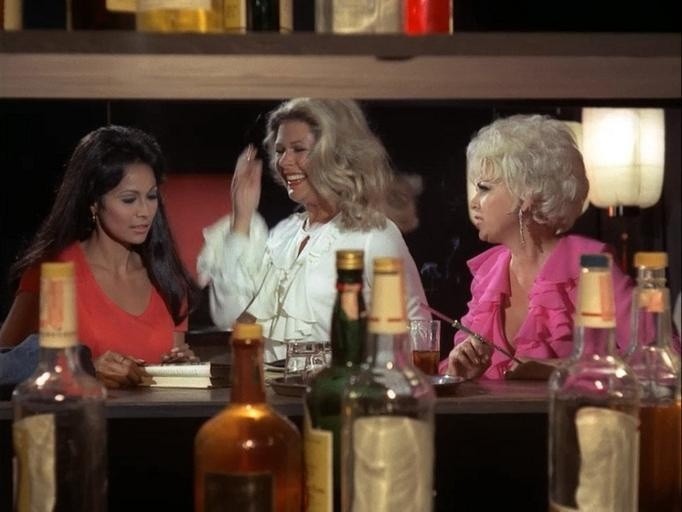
[341,256,432,512]
[301,250,372,512]
[194,324,307,511]
[10,260,105,511]
[618,251,682,406]
[544,250,641,512]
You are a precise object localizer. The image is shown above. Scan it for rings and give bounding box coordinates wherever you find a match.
[120,356,126,363]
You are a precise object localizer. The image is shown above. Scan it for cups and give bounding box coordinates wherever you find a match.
[284,341,333,388]
[412,318,441,378]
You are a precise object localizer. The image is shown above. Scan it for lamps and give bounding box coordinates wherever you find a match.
[583,104,667,221]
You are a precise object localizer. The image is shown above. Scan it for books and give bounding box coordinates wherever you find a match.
[134,360,231,379]
[137,374,229,390]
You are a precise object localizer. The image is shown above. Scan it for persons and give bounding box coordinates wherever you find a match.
[0,125,198,388]
[196,94,438,371]
[431,113,680,392]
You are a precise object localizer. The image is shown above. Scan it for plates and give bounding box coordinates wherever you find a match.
[138,360,234,390]
